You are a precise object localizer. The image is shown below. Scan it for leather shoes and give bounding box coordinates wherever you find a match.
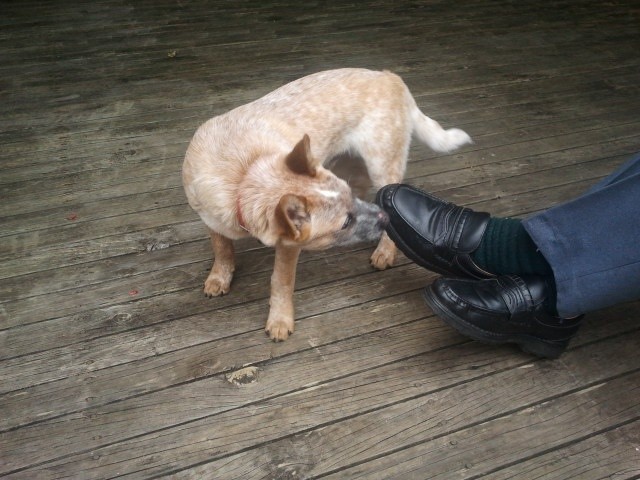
[424,274,584,359]
[375,183,499,281]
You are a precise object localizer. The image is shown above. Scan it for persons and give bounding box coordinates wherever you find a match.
[374,151,639,360]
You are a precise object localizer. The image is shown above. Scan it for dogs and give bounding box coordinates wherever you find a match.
[182,67,475,343]
